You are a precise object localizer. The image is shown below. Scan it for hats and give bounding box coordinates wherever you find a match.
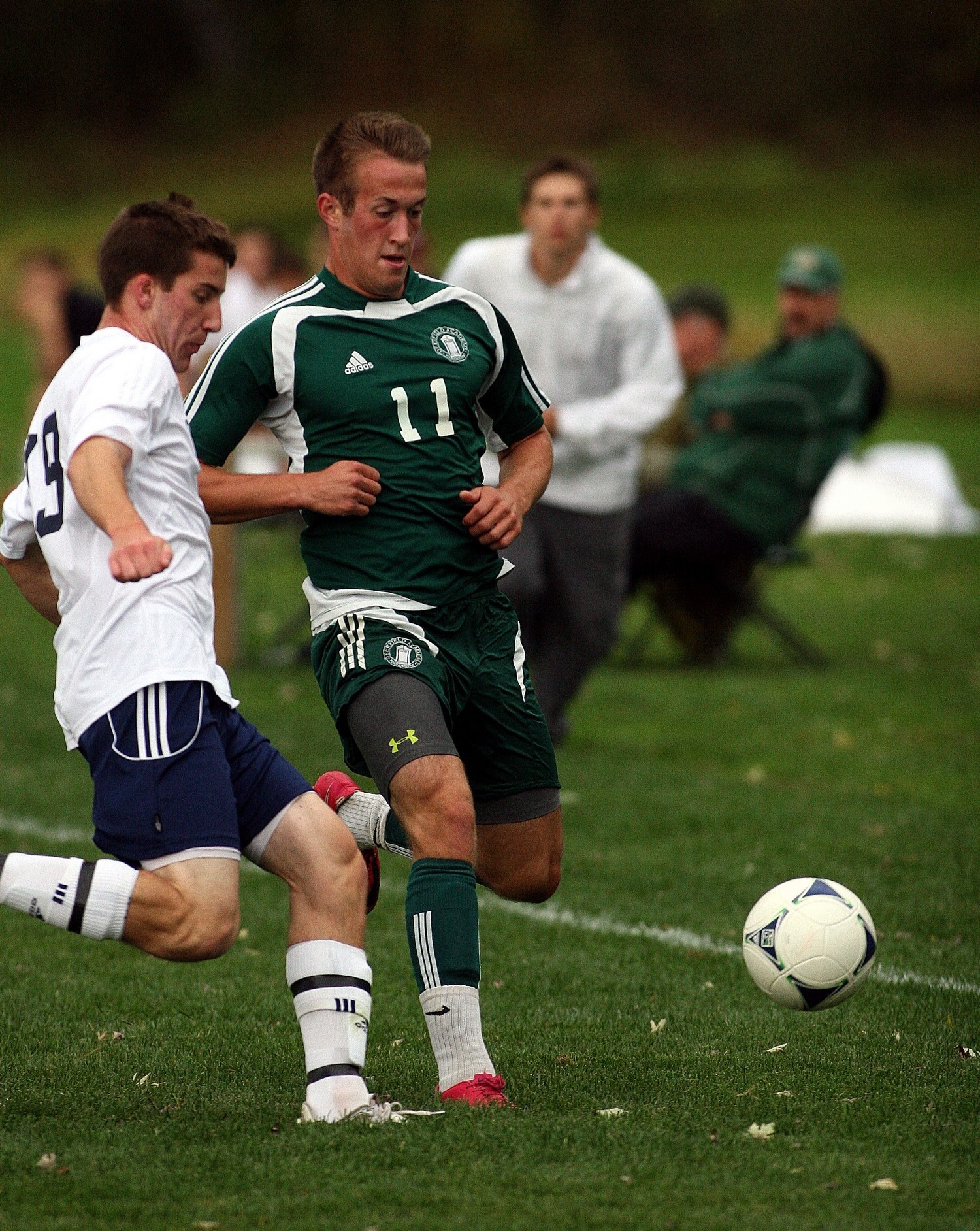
[776,247,840,290]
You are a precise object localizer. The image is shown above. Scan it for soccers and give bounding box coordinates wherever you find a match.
[744,878,881,1011]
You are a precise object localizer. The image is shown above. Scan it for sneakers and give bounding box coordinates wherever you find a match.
[312,770,380,916]
[435,1068,518,1110]
[296,1092,445,1127]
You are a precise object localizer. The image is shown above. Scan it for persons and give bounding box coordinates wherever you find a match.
[0,192,448,1125]
[440,153,686,739]
[631,248,893,661]
[21,238,105,377]
[190,215,304,674]
[185,103,567,1103]
